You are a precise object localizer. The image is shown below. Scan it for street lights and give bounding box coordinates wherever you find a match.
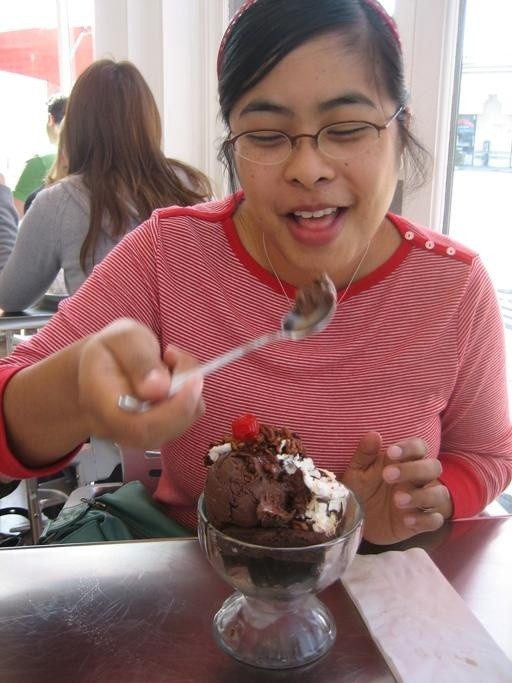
[38,491,133,544]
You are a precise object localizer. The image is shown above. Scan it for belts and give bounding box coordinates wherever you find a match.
[196,477,365,670]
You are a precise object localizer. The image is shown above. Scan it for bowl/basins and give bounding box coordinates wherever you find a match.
[254,232,372,322]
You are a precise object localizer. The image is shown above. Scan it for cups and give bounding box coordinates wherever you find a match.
[224,104,404,167]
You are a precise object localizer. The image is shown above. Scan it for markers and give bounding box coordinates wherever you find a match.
[202,414,348,589]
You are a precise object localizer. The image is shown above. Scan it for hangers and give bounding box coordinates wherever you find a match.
[117,300,335,413]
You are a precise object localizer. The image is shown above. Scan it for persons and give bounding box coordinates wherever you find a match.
[2,54,217,310]
[2,0,512,547]
[12,94,70,218]
